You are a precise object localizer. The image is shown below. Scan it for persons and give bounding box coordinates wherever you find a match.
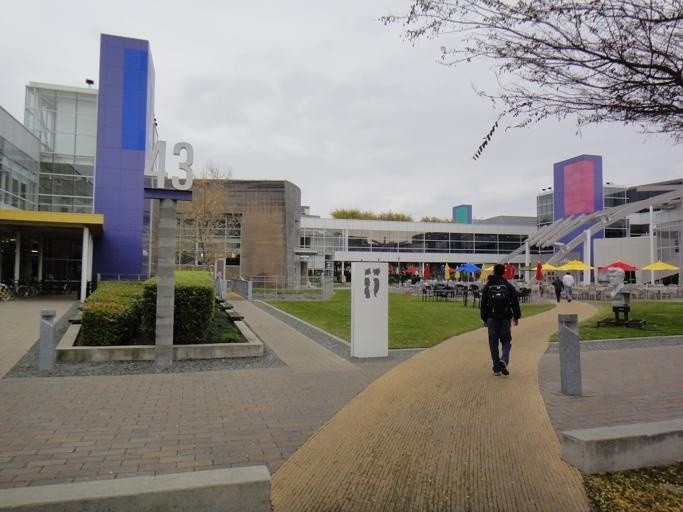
[562,271,574,303]
[551,275,564,302]
[480,264,521,376]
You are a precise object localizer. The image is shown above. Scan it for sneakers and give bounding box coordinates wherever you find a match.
[490,360,508,376]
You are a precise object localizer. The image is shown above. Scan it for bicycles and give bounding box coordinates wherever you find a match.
[0,278,38,301]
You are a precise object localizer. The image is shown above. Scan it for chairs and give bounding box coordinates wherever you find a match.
[422,283,683,308]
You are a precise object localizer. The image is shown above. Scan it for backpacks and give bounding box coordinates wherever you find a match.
[487,283,511,318]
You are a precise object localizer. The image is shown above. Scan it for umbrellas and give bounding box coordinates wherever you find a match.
[405,257,681,287]
